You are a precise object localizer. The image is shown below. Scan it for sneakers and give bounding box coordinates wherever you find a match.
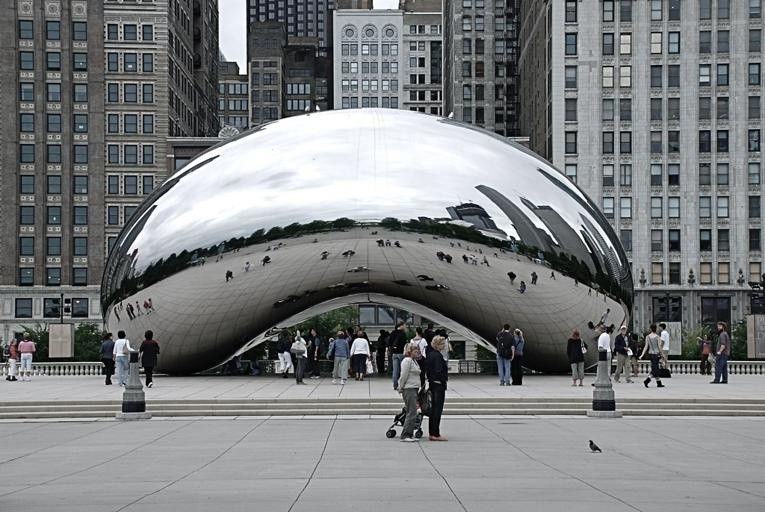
[296,372,363,385]
[399,435,448,442]
[148,382,153,388]
[6,376,31,381]
[721,381,728,383]
[710,381,720,384]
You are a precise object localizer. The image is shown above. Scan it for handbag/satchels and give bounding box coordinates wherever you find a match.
[291,342,305,354]
[123,344,129,355]
[581,347,588,355]
[654,369,671,378]
[329,349,335,360]
[655,354,661,360]
[366,360,374,375]
[503,348,512,360]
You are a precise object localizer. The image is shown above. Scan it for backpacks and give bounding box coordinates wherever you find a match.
[412,338,425,361]
[391,331,404,353]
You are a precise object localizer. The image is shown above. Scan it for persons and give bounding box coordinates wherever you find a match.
[200,224,411,308]
[113,331,135,386]
[218,323,454,441]
[99,333,115,385]
[567,330,588,385]
[18,332,36,381]
[5,339,19,381]
[114,297,154,320]
[139,330,160,388]
[591,327,612,386]
[614,324,670,387]
[696,334,712,374]
[419,234,610,327]
[497,324,516,385]
[709,322,731,383]
[510,329,524,385]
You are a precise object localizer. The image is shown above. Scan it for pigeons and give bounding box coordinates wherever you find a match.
[589,439,601,452]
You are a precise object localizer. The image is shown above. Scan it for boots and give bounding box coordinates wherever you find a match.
[657,381,664,387]
[644,377,651,388]
